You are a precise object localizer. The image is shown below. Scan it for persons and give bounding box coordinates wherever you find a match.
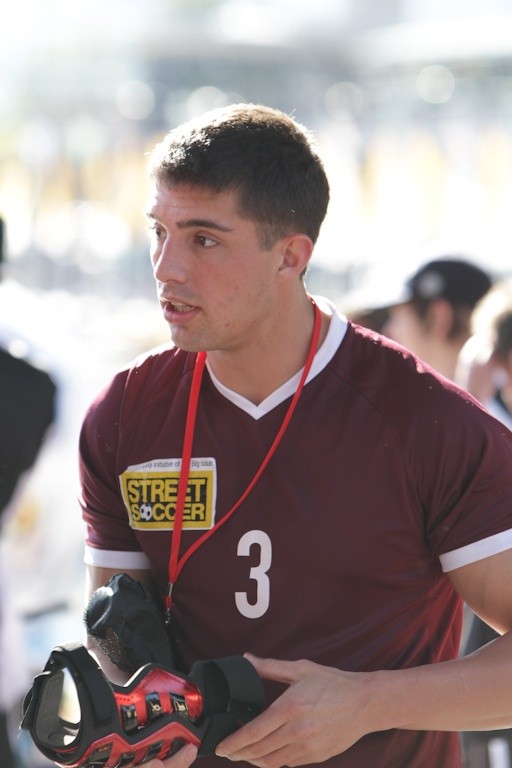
[351,254,512,767]
[76,103,512,768]
[1,219,60,768]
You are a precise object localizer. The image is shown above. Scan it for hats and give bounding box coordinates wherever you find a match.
[372,260,490,323]
